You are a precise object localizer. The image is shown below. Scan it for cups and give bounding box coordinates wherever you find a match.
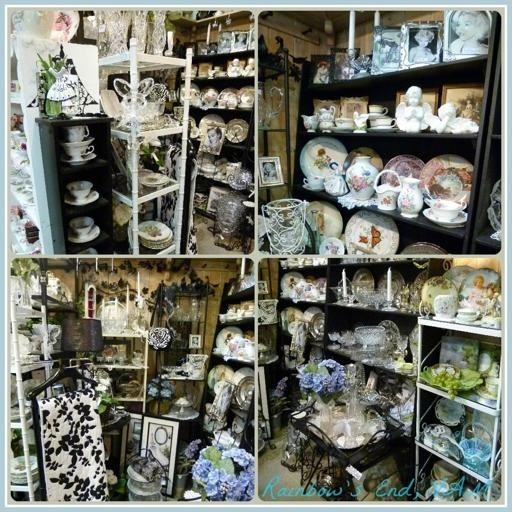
[304,174,325,189]
[367,104,388,114]
[59,124,96,159]
[422,196,467,220]
[376,116,398,128]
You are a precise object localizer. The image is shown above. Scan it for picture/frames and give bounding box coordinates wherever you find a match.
[311,11,492,129]
[258,156,286,188]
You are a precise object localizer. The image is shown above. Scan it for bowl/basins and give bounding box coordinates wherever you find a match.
[65,180,95,199]
[26,221,40,243]
[334,117,354,127]
[457,308,479,321]
[431,362,460,380]
[69,215,93,233]
[484,376,499,394]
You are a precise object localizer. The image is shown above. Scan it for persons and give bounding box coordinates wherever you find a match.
[447,11,490,56]
[467,276,502,311]
[409,25,438,62]
[313,61,332,84]
[262,160,280,182]
[203,127,222,151]
[394,85,432,134]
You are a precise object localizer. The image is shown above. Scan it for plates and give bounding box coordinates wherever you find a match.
[278,270,327,302]
[137,167,168,188]
[11,374,47,422]
[473,389,499,401]
[182,112,248,146]
[214,326,243,353]
[330,125,354,134]
[10,455,39,485]
[435,397,468,429]
[65,225,101,243]
[207,364,255,433]
[95,301,127,334]
[298,200,447,256]
[378,319,400,344]
[228,167,252,191]
[176,80,254,110]
[367,126,399,133]
[138,219,174,249]
[279,305,325,341]
[302,184,326,191]
[300,137,474,207]
[423,208,469,229]
[350,265,501,318]
[59,137,97,165]
[64,189,99,207]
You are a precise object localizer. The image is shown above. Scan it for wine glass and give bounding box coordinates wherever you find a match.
[333,286,394,309]
[326,324,408,369]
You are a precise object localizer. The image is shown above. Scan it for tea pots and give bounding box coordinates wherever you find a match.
[219,304,254,324]
[397,173,423,218]
[300,105,336,135]
[433,294,457,318]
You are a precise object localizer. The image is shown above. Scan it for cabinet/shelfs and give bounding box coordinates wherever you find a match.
[288,9,500,253]
[13,20,254,257]
[257,34,316,253]
[13,262,257,502]
[469,9,500,251]
[259,258,504,502]
